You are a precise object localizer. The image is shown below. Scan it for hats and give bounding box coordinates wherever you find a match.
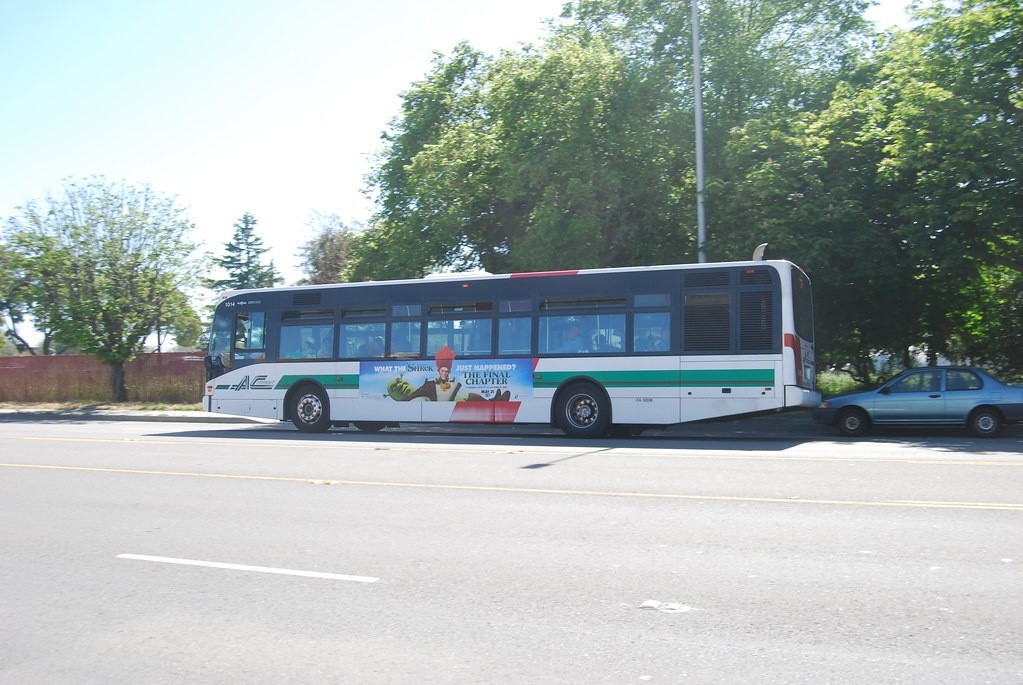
[303,338,315,344]
[567,326,580,333]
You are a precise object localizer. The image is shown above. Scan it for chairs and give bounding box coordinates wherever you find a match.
[950,376,968,390]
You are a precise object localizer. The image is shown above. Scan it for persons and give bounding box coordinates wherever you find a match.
[950,376,969,389]
[237,330,384,358]
[550,327,668,351]
[927,374,941,391]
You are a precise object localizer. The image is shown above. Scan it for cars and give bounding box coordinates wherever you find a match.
[813,365,1023,439]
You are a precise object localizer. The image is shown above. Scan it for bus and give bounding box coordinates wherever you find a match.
[199,243,824,441]
